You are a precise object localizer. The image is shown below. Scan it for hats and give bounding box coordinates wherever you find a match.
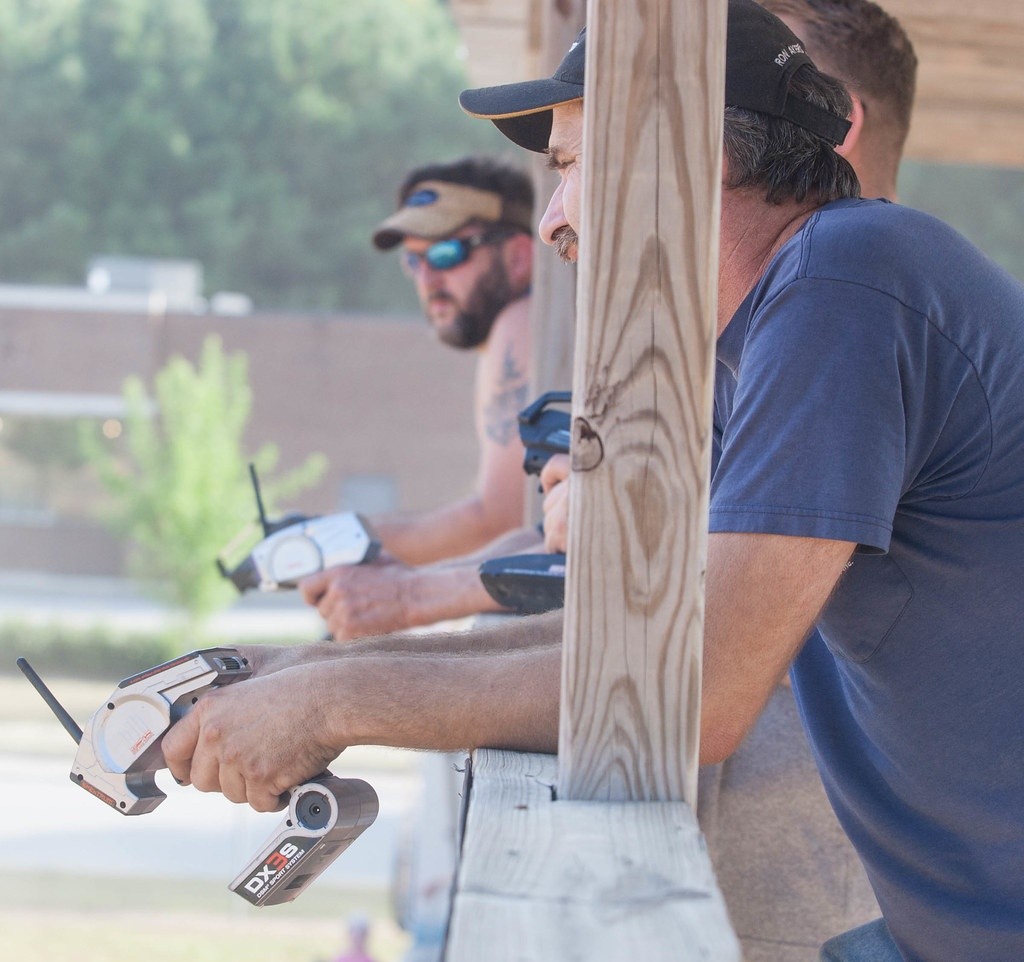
[457,0,852,154]
[372,181,531,249]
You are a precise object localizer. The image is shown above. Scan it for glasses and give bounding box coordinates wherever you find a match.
[404,229,521,271]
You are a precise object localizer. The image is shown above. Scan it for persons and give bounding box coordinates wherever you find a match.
[160,0,1024,962]
